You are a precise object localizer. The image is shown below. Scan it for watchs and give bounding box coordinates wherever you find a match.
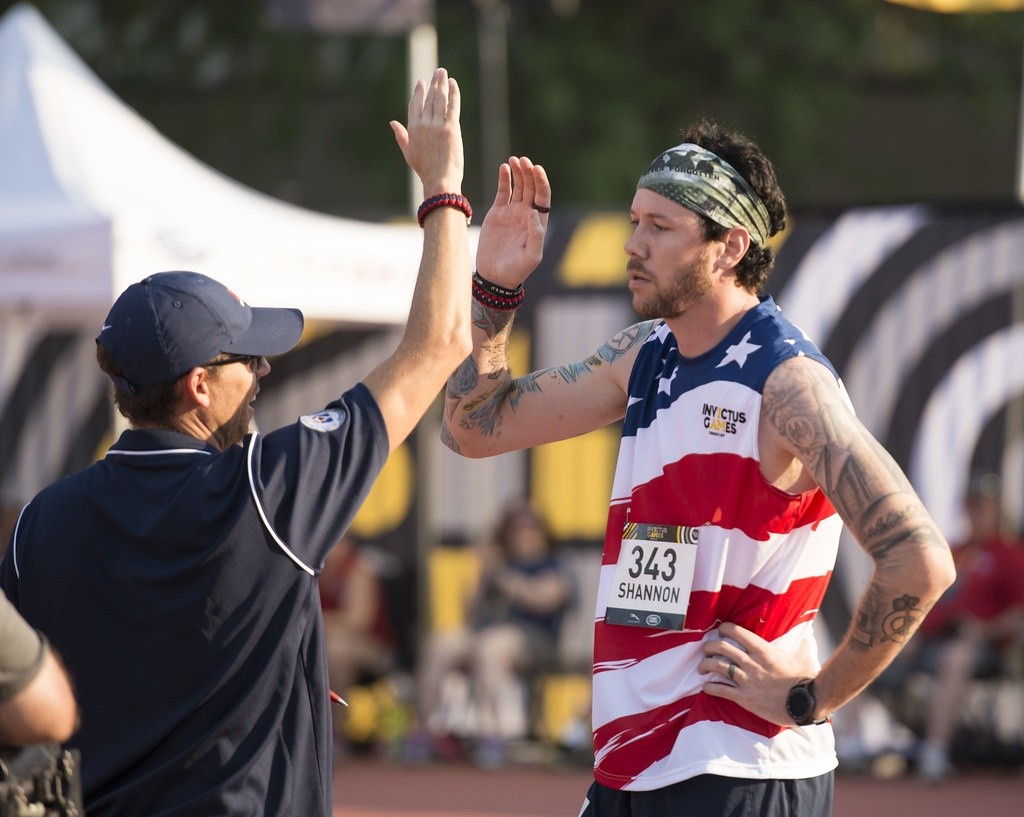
[785,677,825,727]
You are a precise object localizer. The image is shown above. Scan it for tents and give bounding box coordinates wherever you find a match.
[4,0,482,326]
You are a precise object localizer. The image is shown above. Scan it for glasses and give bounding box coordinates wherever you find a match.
[175,355,260,373]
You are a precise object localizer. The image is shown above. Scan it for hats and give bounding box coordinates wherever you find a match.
[95,270,304,396]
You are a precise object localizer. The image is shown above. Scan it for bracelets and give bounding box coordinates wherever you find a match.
[417,193,472,228]
[471,272,524,310]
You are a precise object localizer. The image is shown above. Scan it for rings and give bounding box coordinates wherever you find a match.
[727,663,736,679]
[532,201,550,213]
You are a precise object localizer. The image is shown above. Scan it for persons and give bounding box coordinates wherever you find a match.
[431,503,574,765]
[2,63,474,817]
[2,584,77,817]
[442,123,954,816]
[319,535,390,760]
[877,476,1023,785]
